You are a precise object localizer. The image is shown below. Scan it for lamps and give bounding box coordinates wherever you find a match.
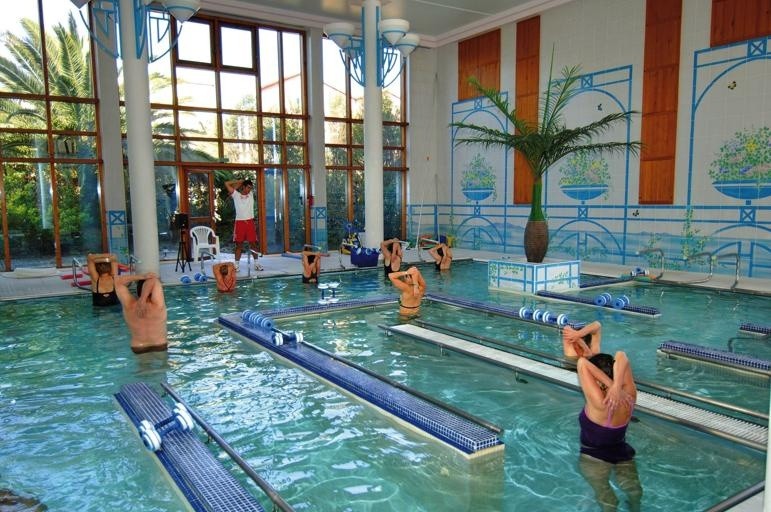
[71,0,202,63]
[323,17,421,89]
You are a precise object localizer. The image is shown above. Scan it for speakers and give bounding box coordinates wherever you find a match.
[175,213,188,230]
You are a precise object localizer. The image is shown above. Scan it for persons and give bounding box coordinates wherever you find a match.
[389,266,426,315]
[429,242,453,270]
[225,178,265,272]
[87,252,120,307]
[114,272,168,353]
[380,238,403,278]
[302,251,331,283]
[562,319,602,360]
[577,351,644,512]
[213,262,237,291]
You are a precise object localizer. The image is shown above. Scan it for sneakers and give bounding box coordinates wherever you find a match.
[235,264,240,272]
[255,264,264,271]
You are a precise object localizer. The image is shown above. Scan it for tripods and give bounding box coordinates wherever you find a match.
[175,229,191,273]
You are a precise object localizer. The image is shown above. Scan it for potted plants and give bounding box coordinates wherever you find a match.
[447,41,645,262]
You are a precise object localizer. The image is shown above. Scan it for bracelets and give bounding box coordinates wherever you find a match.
[405,271,408,275]
[105,257,110,263]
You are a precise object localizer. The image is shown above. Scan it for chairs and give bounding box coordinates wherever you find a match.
[190,226,221,264]
